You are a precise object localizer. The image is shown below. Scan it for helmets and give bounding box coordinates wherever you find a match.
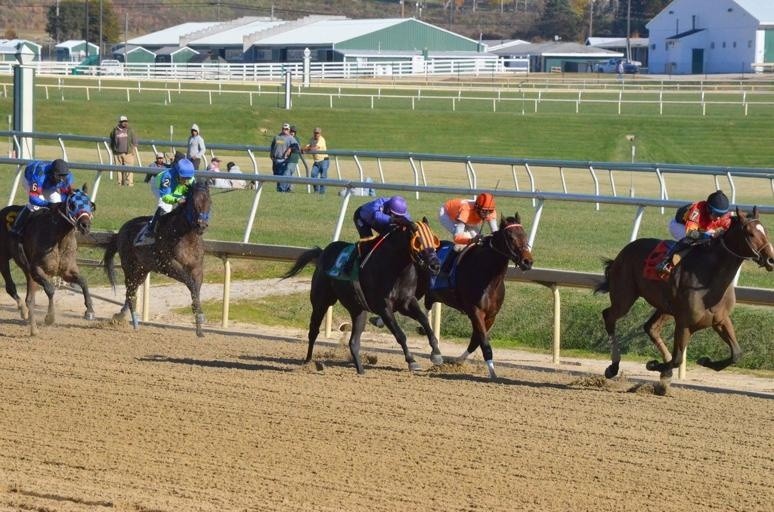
[173,158,195,178]
[389,195,407,216]
[707,189,730,216]
[476,192,496,211]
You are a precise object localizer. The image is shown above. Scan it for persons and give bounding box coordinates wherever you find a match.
[110,116,136,186]
[342,196,412,276]
[148,158,197,236]
[301,128,329,194]
[439,193,498,272]
[656,190,732,282]
[144,123,299,191]
[10,159,74,234]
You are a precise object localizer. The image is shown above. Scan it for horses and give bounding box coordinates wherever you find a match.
[369,212,533,382]
[95,176,212,337]
[279,217,444,374]
[0,182,95,336]
[593,205,774,386]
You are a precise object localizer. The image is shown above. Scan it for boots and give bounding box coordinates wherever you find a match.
[144,206,166,238]
[439,249,459,279]
[10,206,32,238]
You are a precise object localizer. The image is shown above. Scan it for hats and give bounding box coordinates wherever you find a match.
[118,115,128,122]
[156,152,164,159]
[283,123,297,132]
[211,158,221,162]
